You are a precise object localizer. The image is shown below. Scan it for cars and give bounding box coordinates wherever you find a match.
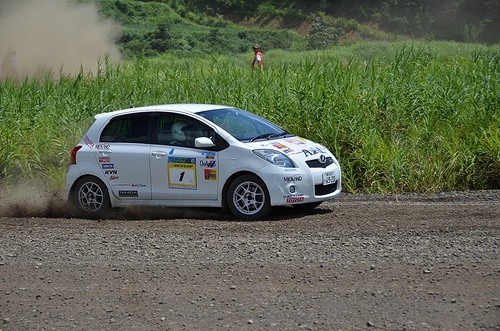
[67,102,341,219]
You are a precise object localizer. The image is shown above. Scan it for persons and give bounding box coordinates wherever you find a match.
[252,44,265,73]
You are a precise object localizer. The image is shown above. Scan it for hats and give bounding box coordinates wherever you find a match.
[253,44,260,48]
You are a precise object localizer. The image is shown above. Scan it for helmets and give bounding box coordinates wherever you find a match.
[171,121,191,141]
